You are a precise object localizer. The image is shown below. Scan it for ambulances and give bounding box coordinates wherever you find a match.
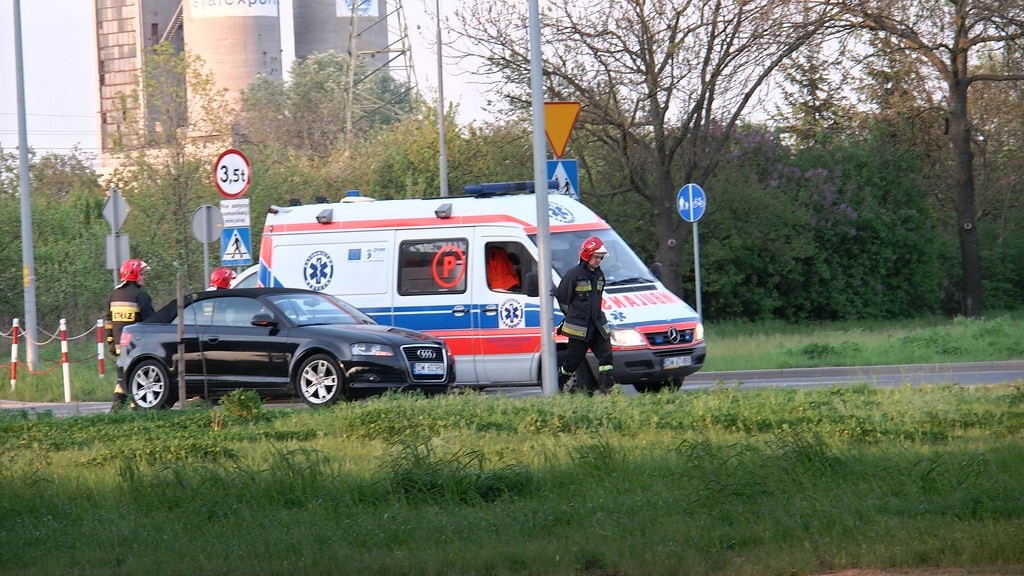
[257,181,706,394]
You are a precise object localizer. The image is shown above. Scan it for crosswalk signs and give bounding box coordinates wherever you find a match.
[545,160,579,203]
[219,230,252,266]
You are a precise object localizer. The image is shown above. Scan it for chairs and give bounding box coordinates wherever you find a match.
[224,307,253,326]
[524,272,538,296]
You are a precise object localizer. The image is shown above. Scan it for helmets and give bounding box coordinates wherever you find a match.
[119,258,148,282]
[210,267,238,289]
[579,237,608,261]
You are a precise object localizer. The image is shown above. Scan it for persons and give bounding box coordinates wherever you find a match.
[557,236,614,389]
[199,267,236,317]
[489,247,520,292]
[105,259,155,408]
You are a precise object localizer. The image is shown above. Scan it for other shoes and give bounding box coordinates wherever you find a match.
[110,405,126,412]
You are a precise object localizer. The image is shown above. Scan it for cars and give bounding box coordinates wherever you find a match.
[115,288,456,414]
[230,264,259,290]
